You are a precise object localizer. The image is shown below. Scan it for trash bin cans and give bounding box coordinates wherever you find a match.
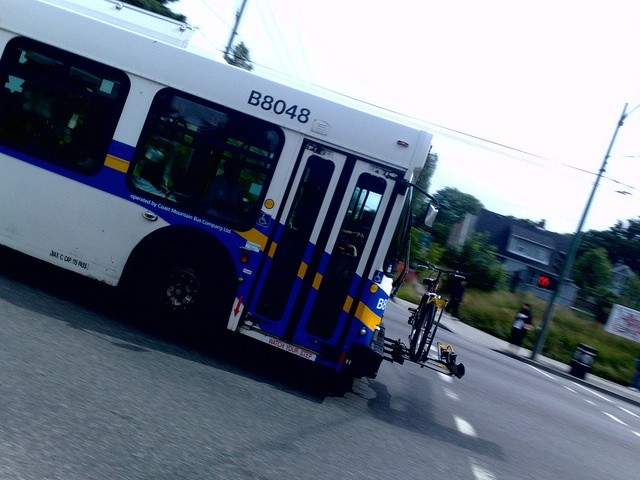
[569,342,598,381]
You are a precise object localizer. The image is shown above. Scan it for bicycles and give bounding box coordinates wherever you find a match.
[406,256,469,362]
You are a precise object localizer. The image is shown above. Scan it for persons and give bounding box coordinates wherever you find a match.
[47,117,96,174]
[509,301,531,344]
[132,136,169,198]
[445,277,469,318]
[210,158,250,198]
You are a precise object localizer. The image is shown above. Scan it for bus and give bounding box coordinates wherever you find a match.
[1,0,443,397]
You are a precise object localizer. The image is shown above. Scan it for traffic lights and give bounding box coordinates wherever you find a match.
[536,273,558,292]
